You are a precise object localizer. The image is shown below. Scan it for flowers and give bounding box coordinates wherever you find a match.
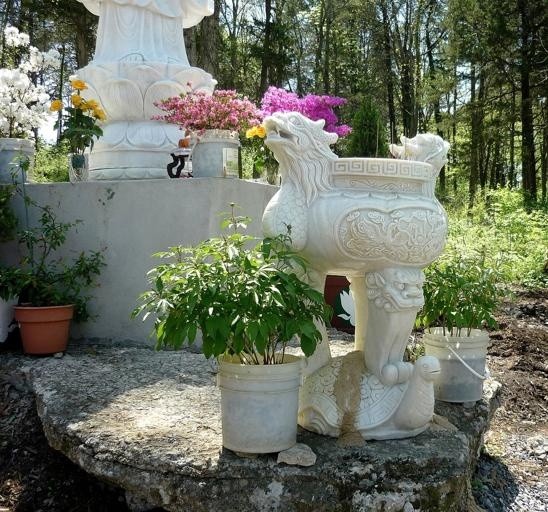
[150,81,263,143]
[256,82,352,166]
[50,79,108,177]
[0,27,62,138]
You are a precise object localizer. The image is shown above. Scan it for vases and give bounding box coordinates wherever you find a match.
[68,153,89,184]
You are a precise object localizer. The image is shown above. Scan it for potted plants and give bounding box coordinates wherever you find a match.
[1,154,109,355]
[131,199,335,457]
[412,244,516,404]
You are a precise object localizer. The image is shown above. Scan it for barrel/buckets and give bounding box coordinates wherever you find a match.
[218,354,302,454]
[422,328,489,403]
